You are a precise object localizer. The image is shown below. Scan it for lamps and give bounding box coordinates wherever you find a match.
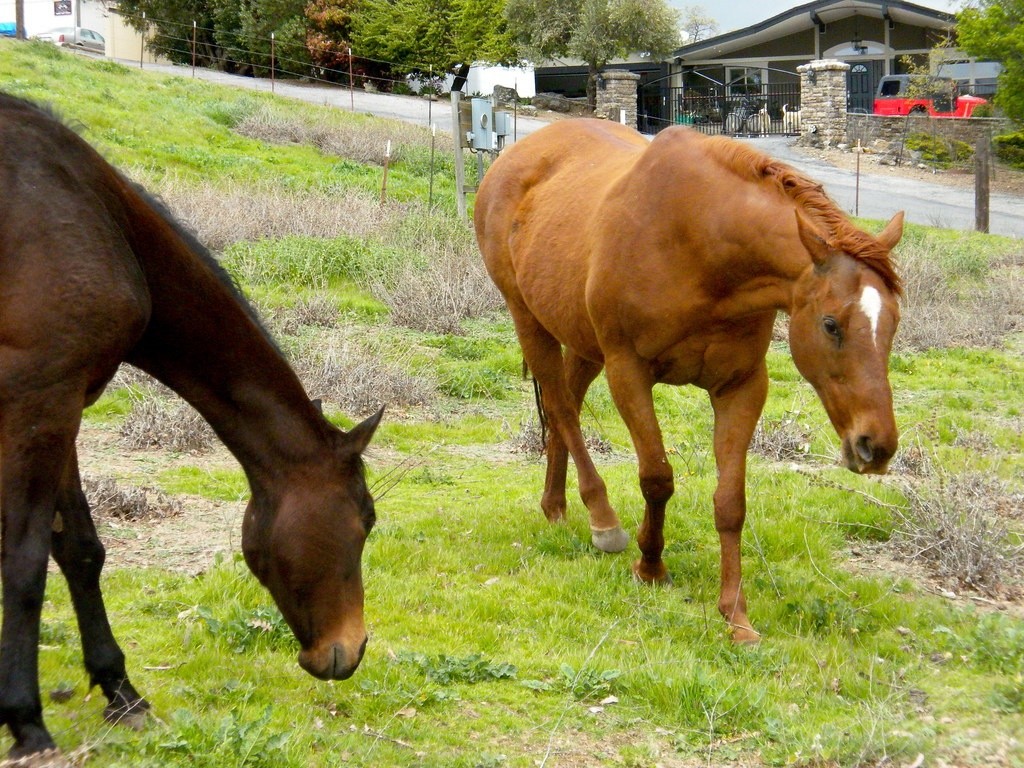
[806,65,816,80]
[597,73,607,90]
[850,9,862,50]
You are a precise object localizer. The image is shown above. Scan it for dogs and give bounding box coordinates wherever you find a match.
[759,103,801,137]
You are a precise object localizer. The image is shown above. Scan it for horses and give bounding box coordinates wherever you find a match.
[1,91,388,765]
[474,117,907,643]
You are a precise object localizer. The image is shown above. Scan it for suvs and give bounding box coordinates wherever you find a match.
[874,73,988,119]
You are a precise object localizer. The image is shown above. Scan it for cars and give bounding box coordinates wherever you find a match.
[35,25,106,55]
